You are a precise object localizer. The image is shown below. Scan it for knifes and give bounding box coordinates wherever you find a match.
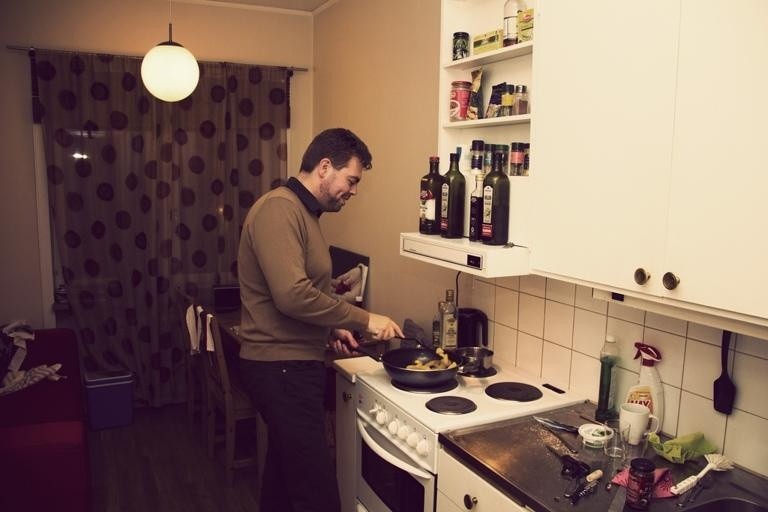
[533,414,578,432]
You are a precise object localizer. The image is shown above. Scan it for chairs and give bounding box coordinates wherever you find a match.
[176,288,209,444]
[192,305,257,490]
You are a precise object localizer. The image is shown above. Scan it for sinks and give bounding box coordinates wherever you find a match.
[681,496,768,512]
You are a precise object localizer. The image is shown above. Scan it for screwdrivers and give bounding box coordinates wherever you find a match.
[545,445,591,476]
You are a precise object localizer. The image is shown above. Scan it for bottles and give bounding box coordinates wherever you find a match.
[442,151,466,240]
[432,301,446,346]
[499,83,529,117]
[503,1,528,48]
[462,139,529,174]
[441,288,459,346]
[468,172,484,242]
[483,153,513,249]
[418,154,442,234]
[593,333,620,425]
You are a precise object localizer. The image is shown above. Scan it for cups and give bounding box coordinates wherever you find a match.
[603,417,632,461]
[617,402,662,446]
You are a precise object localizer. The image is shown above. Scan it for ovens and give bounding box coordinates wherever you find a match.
[351,421,435,512]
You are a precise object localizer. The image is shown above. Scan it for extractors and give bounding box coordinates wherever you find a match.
[399,232,530,278]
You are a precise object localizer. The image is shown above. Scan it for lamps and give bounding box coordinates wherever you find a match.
[139,0,199,103]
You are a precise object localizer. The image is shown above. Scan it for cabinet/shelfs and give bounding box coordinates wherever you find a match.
[534,0,767,328]
[397,0,531,278]
[436,450,530,511]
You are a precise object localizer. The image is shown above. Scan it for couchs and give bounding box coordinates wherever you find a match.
[2,327,90,511]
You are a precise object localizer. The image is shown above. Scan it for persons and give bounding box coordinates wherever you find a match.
[236,127,405,511]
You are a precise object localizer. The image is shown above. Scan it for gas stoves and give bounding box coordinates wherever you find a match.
[354,360,592,434]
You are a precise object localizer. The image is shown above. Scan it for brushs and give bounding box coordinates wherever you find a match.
[669,453,735,496]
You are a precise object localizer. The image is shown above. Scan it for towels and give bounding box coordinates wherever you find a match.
[610,467,674,500]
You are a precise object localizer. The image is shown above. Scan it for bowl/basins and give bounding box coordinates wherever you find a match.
[453,344,496,374]
[578,422,614,449]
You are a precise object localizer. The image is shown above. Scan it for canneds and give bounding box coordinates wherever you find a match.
[450,81,473,121]
[627,459,656,511]
[453,32,468,60]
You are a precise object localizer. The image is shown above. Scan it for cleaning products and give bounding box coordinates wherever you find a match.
[594,334,622,423]
[624,341,666,435]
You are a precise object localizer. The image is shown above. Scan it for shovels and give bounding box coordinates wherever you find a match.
[394,335,444,359]
[713,330,735,415]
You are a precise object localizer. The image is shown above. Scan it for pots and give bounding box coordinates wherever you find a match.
[345,340,463,389]
